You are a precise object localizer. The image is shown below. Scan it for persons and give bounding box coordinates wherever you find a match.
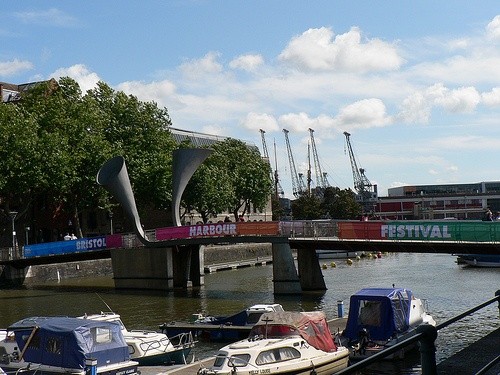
[223,215,244,223]
[64,233,78,240]
[481,206,492,221]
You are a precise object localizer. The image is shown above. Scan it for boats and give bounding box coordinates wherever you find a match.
[0,284,438,375]
[463,259,500,267]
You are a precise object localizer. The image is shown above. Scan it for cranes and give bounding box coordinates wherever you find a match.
[343,132,380,208]
[258,128,284,198]
[286,129,335,200]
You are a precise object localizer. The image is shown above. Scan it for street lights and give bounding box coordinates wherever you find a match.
[10,212,19,262]
[25,227,30,248]
